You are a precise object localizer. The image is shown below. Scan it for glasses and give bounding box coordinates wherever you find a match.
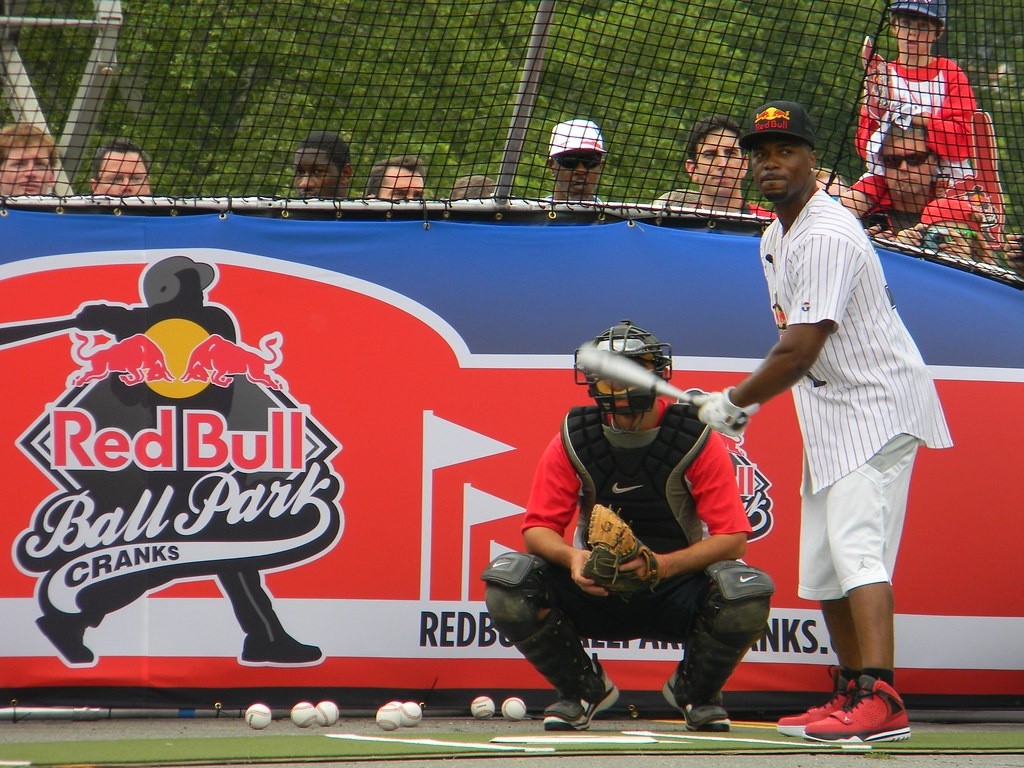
[883,152,932,168]
[551,152,603,169]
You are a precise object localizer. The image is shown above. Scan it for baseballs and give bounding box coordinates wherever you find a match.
[472,695,496,720]
[502,697,526,722]
[401,701,423,726]
[291,701,316,727]
[376,701,402,731]
[245,703,271,730]
[315,701,339,727]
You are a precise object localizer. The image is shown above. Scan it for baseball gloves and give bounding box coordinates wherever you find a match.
[579,503,660,594]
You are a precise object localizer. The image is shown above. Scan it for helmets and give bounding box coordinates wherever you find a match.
[573,321,673,436]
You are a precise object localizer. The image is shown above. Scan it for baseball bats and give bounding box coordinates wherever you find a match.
[578,343,748,432]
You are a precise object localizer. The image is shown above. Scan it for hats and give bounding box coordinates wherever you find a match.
[740,99,816,149]
[886,0,946,27]
[548,120,606,160]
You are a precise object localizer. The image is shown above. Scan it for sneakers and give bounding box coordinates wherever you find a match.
[802,674,912,743]
[543,651,624,729]
[776,664,858,736]
[662,662,729,731]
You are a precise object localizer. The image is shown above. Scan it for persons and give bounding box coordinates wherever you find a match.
[650,113,777,219]
[366,156,425,199]
[478,318,775,734]
[89,138,151,196]
[543,119,607,202]
[293,133,352,197]
[837,0,1024,274]
[0,123,58,197]
[450,176,495,200]
[695,100,954,745]
[814,168,850,201]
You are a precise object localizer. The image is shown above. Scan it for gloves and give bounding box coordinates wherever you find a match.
[692,386,760,438]
